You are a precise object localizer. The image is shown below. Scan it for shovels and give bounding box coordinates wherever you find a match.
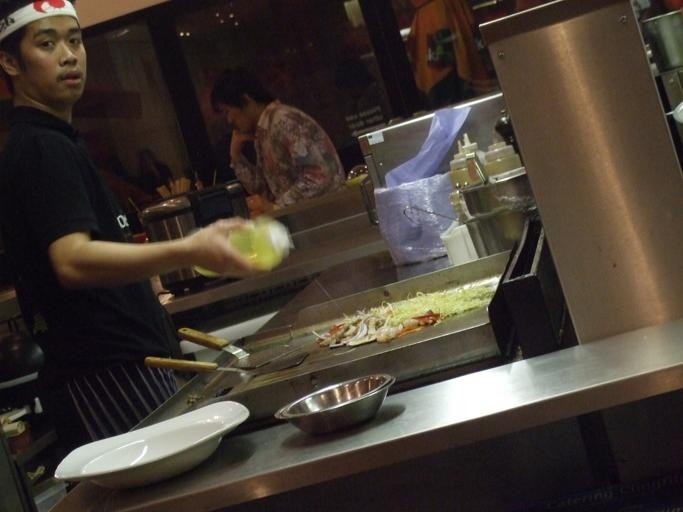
[144,352,309,379]
[177,327,306,369]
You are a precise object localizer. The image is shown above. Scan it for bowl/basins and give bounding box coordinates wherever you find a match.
[272,373,398,432]
[52,400,251,489]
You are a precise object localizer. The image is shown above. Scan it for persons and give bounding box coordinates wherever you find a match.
[0,0,266,453]
[209,66,348,217]
[134,148,173,183]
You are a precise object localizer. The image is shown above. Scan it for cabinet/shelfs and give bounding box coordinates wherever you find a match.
[0,398,74,512]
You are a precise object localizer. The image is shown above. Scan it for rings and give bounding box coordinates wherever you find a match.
[249,199,253,203]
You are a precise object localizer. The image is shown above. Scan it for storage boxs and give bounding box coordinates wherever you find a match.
[31,478,69,511]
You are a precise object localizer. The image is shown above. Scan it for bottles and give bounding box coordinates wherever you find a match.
[190,215,295,279]
[479,137,523,178]
[449,132,485,184]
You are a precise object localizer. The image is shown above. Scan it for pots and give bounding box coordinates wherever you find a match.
[141,183,251,293]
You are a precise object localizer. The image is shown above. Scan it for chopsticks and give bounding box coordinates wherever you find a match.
[155,177,191,198]
